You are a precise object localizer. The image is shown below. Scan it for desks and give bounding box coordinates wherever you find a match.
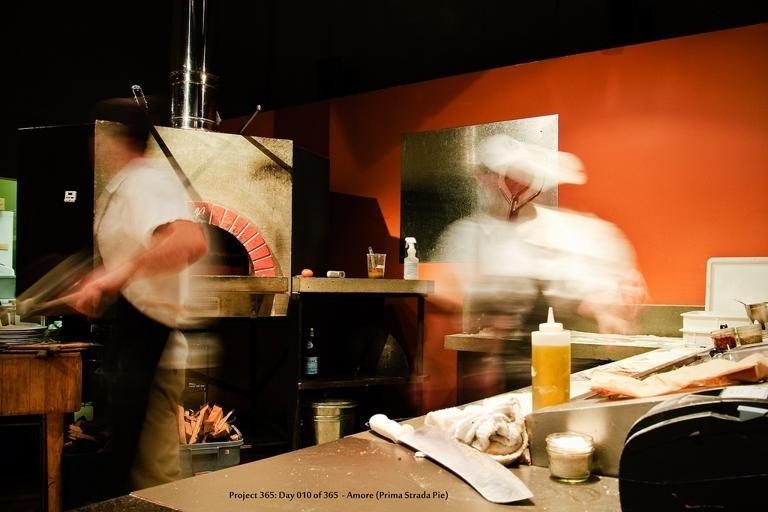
[0,341,93,511]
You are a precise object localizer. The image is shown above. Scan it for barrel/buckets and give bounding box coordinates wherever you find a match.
[312,399,359,446]
[183,440,243,479]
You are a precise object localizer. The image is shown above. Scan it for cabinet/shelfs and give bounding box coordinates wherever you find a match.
[291,279,435,451]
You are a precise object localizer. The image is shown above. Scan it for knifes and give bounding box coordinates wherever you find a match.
[366,412,536,505]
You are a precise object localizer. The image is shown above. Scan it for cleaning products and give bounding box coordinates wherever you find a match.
[402,236,419,281]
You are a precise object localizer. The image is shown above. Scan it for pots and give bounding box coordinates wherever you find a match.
[297,396,357,445]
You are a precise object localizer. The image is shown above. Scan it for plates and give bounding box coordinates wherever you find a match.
[0,323,47,347]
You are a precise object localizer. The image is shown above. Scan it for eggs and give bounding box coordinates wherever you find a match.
[301,268,314,276]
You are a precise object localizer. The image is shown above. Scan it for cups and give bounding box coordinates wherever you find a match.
[367,254,387,278]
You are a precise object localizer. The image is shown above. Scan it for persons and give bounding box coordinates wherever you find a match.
[74,98,210,489]
[430,130,649,401]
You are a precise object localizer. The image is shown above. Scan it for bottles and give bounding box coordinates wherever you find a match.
[305,327,319,378]
[712,319,763,348]
[532,307,572,409]
[547,430,594,484]
